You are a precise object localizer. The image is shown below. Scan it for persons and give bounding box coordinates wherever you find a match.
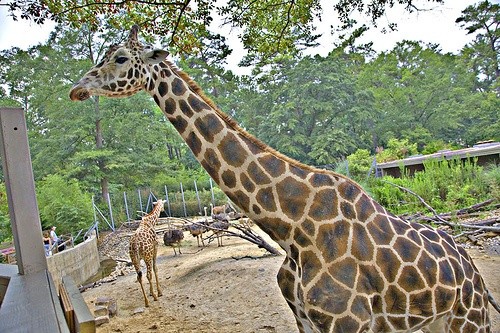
[50,226,57,244]
[42,231,50,255]
[52,235,65,254]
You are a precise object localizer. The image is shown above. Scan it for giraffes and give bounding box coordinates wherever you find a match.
[129,198,167,305]
[69,24,500,332]
